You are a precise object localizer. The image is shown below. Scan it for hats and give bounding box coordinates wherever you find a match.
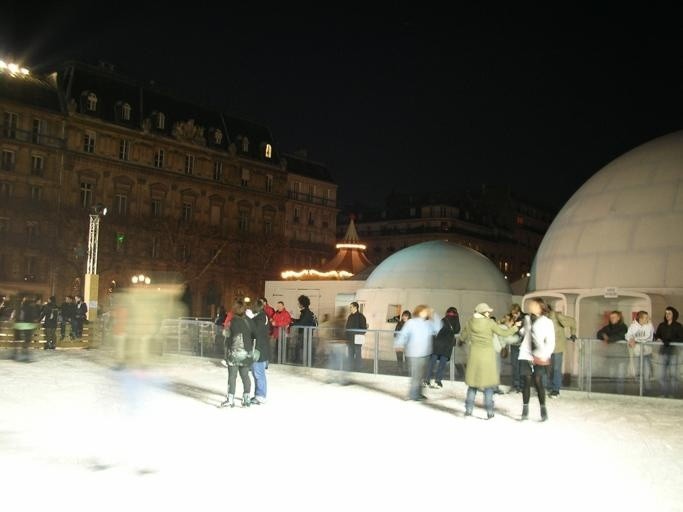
[474,303,495,314]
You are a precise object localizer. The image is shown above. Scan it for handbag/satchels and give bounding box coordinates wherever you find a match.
[226,334,248,363]
[251,348,261,361]
[500,344,510,360]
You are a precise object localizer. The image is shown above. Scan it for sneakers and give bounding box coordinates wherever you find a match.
[464,411,472,415]
[516,413,549,423]
[488,411,496,418]
[423,380,443,387]
[508,384,523,395]
[221,393,261,407]
[413,394,427,401]
[549,390,560,396]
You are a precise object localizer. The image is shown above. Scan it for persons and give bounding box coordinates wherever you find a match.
[343,302,366,371]
[217,301,251,406]
[216,295,317,368]
[624,311,654,398]
[514,297,555,423]
[0,292,158,375]
[653,306,683,399]
[385,297,578,419]
[245,300,269,404]
[594,311,628,395]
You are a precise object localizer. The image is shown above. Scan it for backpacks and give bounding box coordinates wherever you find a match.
[311,312,319,330]
